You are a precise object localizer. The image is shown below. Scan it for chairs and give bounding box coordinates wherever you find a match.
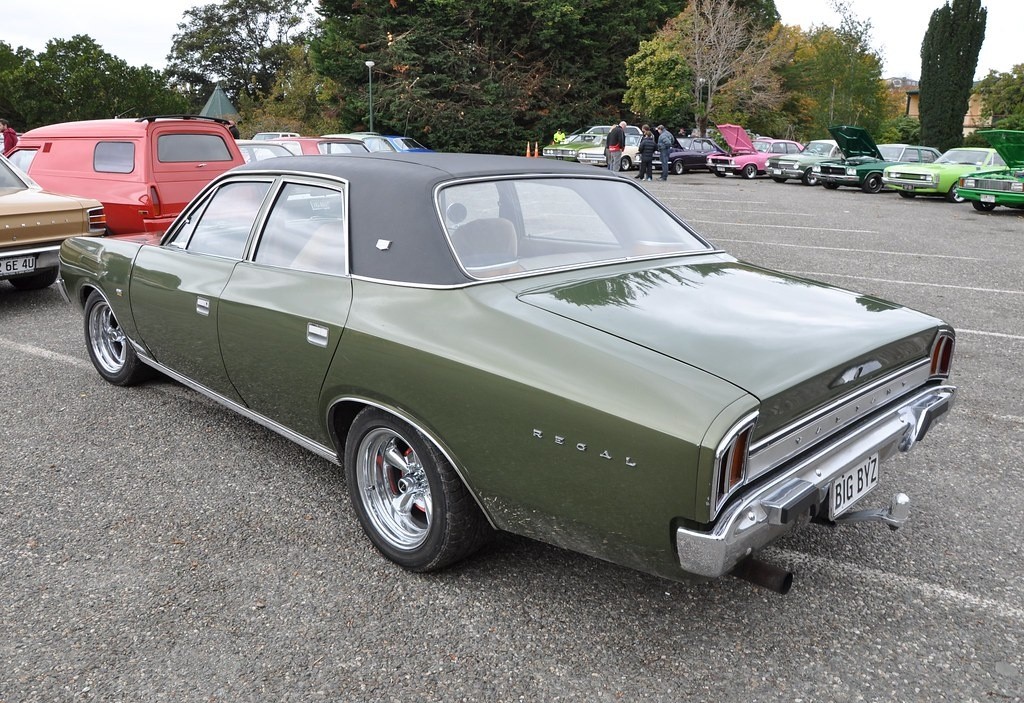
[449,217,518,267]
[291,222,344,276]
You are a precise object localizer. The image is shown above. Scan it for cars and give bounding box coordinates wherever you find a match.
[706,124,805,179]
[543,132,608,162]
[635,136,733,175]
[352,135,434,152]
[577,134,640,172]
[957,128,1024,212]
[271,137,370,155]
[1,153,106,290]
[234,139,342,213]
[763,138,844,187]
[54,151,959,596]
[810,124,953,193]
[882,147,1009,204]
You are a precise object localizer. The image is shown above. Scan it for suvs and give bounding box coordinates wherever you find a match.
[562,126,643,143]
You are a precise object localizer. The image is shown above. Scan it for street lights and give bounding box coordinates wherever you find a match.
[366,61,376,132]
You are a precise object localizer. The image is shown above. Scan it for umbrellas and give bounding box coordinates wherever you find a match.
[196,84,244,124]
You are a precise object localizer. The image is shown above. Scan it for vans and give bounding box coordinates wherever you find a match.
[6,114,248,235]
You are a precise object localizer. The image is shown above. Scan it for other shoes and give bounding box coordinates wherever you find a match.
[648,178,652,181]
[656,177,666,181]
[634,175,644,178]
[643,178,648,181]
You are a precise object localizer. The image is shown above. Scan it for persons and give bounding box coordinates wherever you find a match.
[228,120,239,139]
[609,121,627,171]
[0,119,18,155]
[638,132,658,182]
[553,129,565,144]
[605,125,616,170]
[655,125,674,181]
[634,124,654,180]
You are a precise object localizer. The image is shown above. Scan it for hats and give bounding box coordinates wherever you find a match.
[556,128,561,131]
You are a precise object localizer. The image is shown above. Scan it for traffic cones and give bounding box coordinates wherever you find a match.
[533,142,539,157]
[525,142,531,157]
